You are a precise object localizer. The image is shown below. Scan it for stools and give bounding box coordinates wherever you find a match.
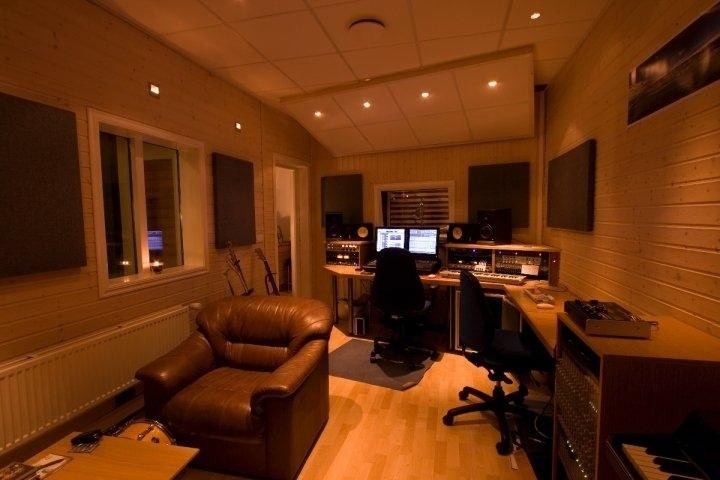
[284,259,292,292]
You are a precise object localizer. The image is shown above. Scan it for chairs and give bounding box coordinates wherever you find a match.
[366,246,441,377]
[446,267,552,456]
[134,295,334,480]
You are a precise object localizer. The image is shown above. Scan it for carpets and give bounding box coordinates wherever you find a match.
[512,395,570,480]
[326,338,441,390]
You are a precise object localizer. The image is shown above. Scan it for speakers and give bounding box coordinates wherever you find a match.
[449,223,479,245]
[326,212,345,239]
[353,223,374,240]
[477,208,512,244]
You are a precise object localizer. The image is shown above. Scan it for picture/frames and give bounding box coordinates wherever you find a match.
[625,0,720,129]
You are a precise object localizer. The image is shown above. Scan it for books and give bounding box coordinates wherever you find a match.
[564,301,651,339]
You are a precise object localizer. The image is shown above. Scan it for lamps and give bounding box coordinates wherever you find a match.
[345,15,390,46]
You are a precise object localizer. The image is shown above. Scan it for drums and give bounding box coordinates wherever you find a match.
[111,417,176,445]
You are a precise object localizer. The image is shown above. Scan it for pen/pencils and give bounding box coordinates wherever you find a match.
[36,459,64,471]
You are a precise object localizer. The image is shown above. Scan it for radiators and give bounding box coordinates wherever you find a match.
[0,306,194,455]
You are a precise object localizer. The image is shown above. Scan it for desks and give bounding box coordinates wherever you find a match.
[18,430,201,479]
[322,239,577,444]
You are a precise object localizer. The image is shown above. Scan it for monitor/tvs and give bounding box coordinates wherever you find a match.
[407,227,440,261]
[147,228,163,251]
[375,227,407,260]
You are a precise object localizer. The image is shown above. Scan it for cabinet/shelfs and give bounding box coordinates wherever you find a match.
[278,241,291,291]
[551,305,719,480]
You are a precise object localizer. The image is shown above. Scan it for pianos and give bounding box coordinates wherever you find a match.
[439,269,527,285]
[606,409,720,480]
